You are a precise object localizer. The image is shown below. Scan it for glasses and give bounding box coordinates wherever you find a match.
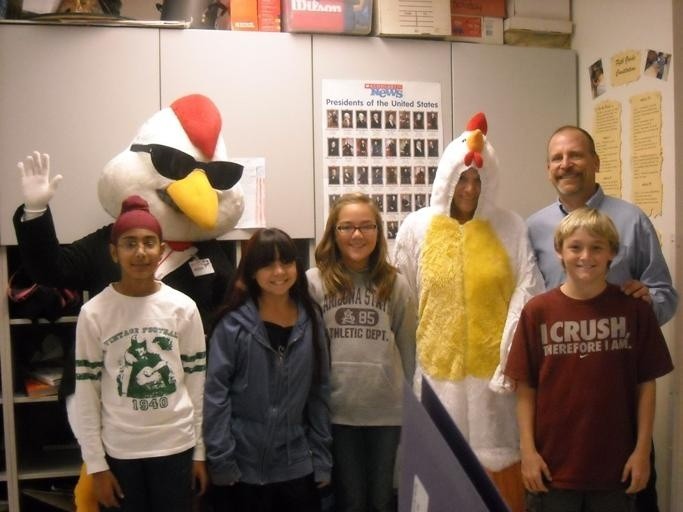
[115,239,160,249]
[336,223,376,233]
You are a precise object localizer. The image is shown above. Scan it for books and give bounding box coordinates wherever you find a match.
[26,365,64,400]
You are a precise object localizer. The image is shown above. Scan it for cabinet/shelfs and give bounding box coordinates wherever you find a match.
[1,248,92,511]
[0,24,312,242]
[314,35,577,241]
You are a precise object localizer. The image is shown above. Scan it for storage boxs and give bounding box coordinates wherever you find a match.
[227,1,575,47]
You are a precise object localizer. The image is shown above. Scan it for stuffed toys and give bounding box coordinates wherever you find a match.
[11,92,245,512]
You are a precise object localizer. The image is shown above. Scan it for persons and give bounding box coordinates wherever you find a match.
[526,125,678,511]
[390,111,548,512]
[502,206,675,511]
[328,110,438,239]
[203,229,334,512]
[76,194,209,511]
[305,194,417,512]
[591,63,606,97]
[643,49,671,81]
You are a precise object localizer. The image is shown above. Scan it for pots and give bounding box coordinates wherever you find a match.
[155,0,229,30]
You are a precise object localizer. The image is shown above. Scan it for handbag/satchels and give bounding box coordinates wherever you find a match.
[7,271,83,326]
